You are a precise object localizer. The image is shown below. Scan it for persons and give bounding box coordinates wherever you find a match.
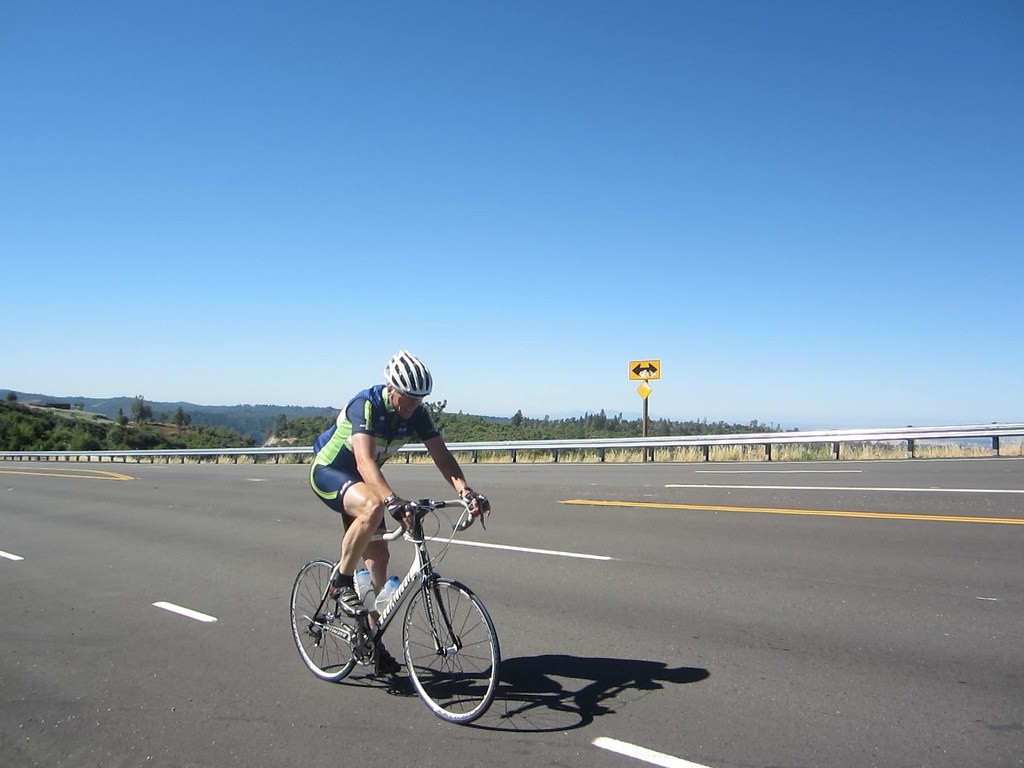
[310,349,490,677]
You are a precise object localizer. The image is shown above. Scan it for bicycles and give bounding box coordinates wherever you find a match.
[289,493,500,725]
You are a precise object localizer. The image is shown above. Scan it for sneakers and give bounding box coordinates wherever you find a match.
[369,640,401,672]
[329,582,370,615]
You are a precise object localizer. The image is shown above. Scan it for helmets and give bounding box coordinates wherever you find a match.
[383,350,433,400]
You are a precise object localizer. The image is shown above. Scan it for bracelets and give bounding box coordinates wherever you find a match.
[459,487,470,499]
[384,494,398,506]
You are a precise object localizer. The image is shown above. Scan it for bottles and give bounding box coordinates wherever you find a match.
[357,567,375,612]
[374,576,401,615]
